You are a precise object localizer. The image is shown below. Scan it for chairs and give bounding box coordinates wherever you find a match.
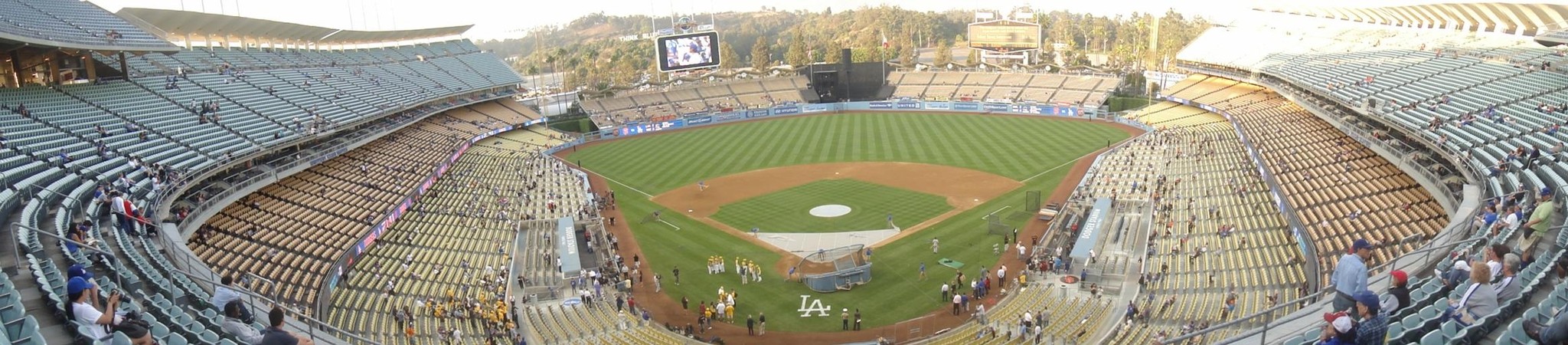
[1433,269,1444,283]
[1,0,721,345]
[728,28,1568,344]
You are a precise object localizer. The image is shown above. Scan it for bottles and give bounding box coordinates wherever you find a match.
[1390,270,1408,283]
[1455,259,1471,272]
[1540,188,1551,195]
[1324,311,1352,334]
[1353,239,1374,249]
[1352,290,1379,307]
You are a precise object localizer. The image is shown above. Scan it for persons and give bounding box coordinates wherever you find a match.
[1,29,1567,345]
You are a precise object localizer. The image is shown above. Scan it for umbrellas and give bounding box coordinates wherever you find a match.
[1522,320,1543,345]
